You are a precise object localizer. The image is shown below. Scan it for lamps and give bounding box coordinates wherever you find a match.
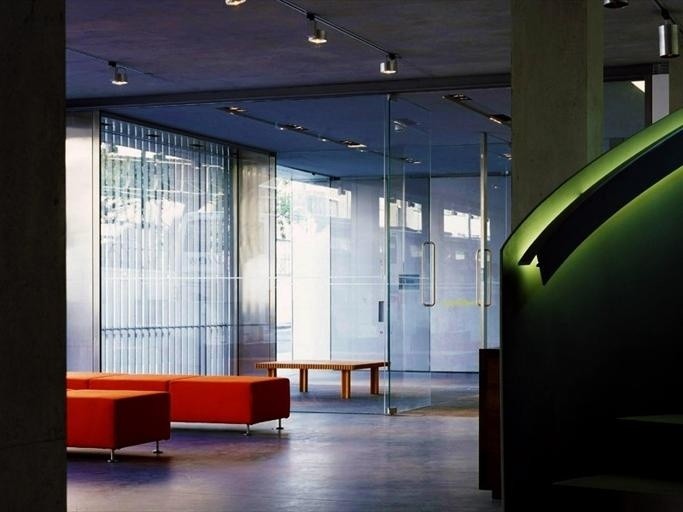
[110,66,129,87]
[309,16,400,76]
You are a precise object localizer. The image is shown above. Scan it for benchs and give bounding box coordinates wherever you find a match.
[68,366,293,467]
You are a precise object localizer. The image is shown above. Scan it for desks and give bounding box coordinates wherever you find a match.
[258,357,389,399]
